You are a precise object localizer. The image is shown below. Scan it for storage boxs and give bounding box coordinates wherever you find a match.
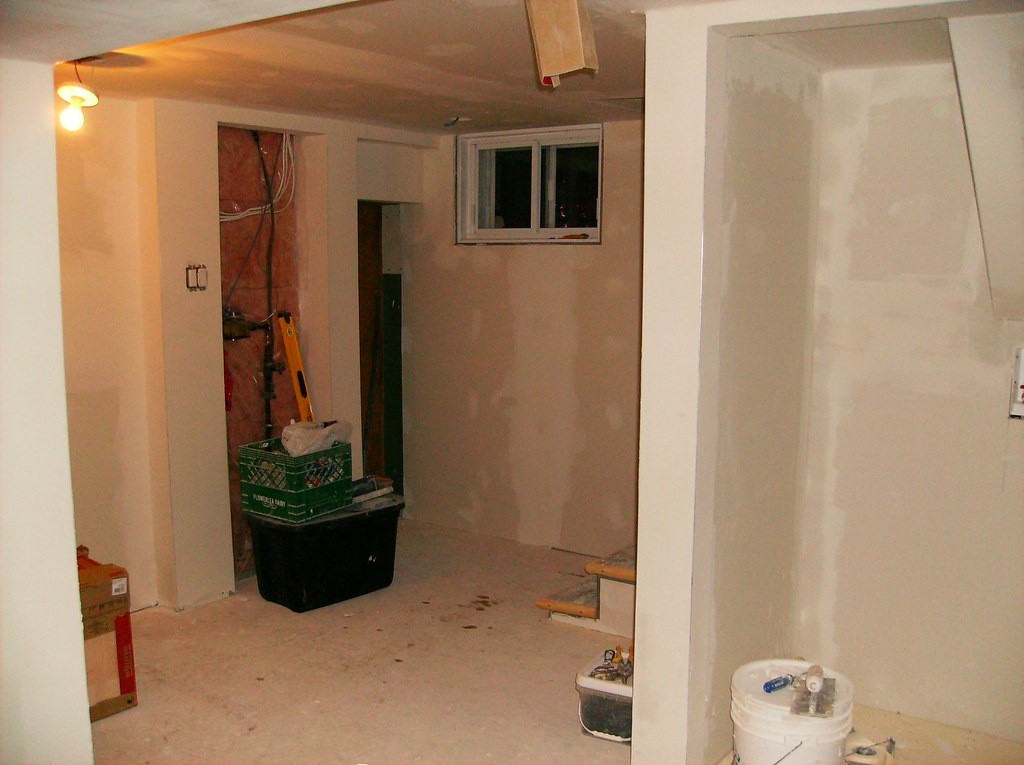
[237,435,353,523]
[575,649,633,744]
[76,544,137,723]
[242,490,406,613]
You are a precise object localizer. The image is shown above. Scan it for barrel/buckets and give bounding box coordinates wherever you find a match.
[730,658,854,765]
